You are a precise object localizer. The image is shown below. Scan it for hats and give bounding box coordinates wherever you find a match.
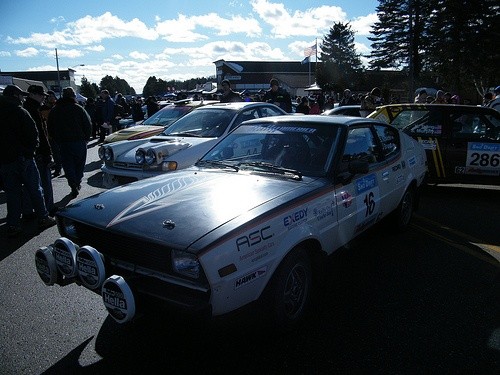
[27,85,49,95]
[3,85,29,95]
[221,79,230,87]
[63,88,73,98]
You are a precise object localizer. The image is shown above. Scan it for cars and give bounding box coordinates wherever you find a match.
[97,88,378,191]
[34,114,429,334]
[357,103,500,199]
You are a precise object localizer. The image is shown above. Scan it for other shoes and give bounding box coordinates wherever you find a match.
[54,170,61,176]
[49,207,65,217]
[38,219,56,230]
[7,227,22,236]
[22,213,39,224]
[77,185,81,190]
[72,189,79,195]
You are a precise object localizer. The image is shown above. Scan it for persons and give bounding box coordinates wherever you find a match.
[335,87,399,117]
[220,79,242,103]
[22,85,64,223]
[410,89,461,133]
[291,93,334,115]
[0,84,56,237]
[74,89,132,143]
[240,79,292,113]
[41,88,92,198]
[473,85,500,134]
[131,92,218,124]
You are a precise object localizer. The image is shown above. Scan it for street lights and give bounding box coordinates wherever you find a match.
[58,63,85,90]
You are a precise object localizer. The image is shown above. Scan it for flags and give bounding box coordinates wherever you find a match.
[301,57,309,64]
[304,44,317,56]
